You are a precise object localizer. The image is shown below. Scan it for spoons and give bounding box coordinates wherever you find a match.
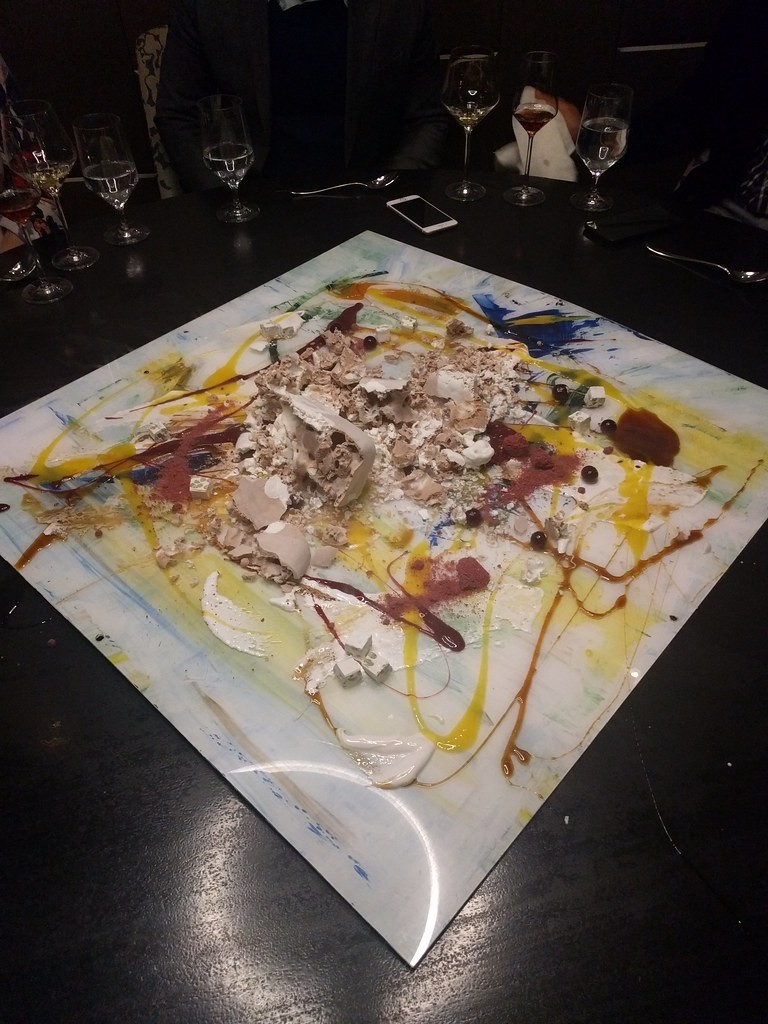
[646,241,768,284]
[288,171,400,194]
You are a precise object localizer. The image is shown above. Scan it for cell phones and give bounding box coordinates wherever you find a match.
[386,194,458,234]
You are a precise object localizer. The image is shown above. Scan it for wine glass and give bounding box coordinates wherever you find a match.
[568,83,635,213]
[441,44,500,202]
[71,112,150,247]
[0,154,73,306]
[502,50,559,207]
[198,94,260,225]
[0,99,99,270]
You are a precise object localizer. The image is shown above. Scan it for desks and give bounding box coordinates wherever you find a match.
[0,176,768,1024]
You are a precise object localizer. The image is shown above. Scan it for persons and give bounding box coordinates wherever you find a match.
[154,0,451,195]
[0,52,68,253]
